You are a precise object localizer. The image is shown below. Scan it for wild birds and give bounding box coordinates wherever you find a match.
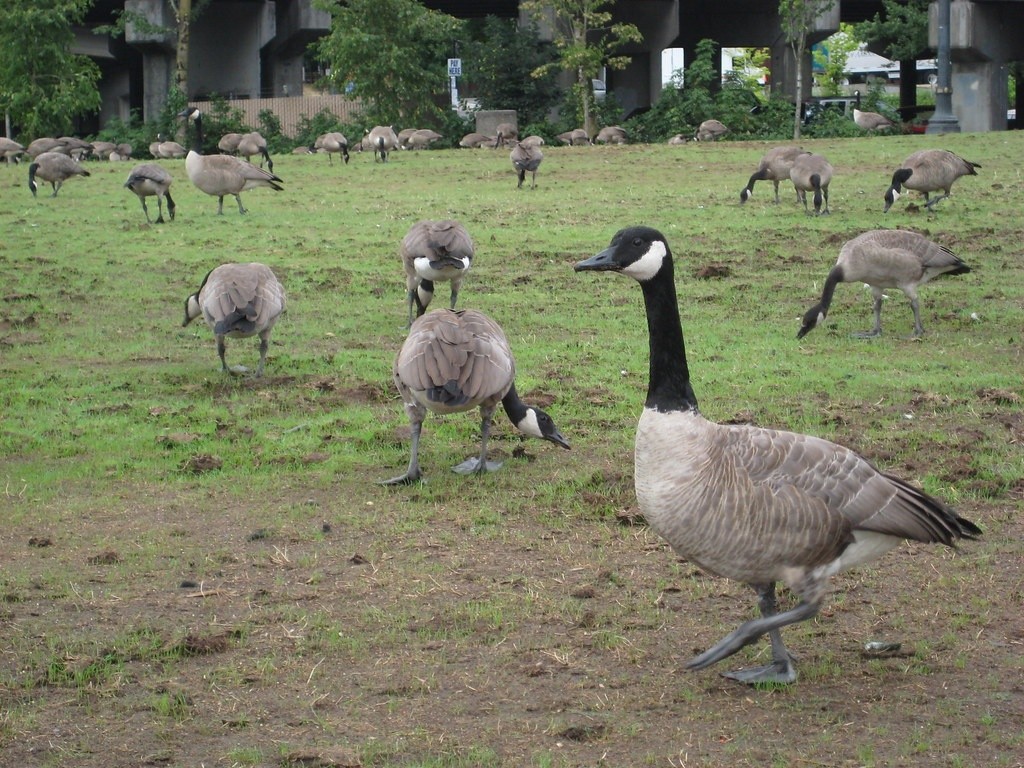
[216,132,274,173]
[853,90,897,136]
[511,135,544,189]
[123,161,175,225]
[796,229,972,344]
[400,219,473,331]
[22,136,132,162]
[462,122,629,150]
[573,227,984,686]
[29,152,90,199]
[181,261,287,379]
[669,120,731,145]
[179,108,284,217]
[791,152,834,216]
[0,137,28,168]
[149,133,188,160]
[739,145,811,204]
[306,124,443,165]
[881,150,981,216]
[372,309,571,488]
